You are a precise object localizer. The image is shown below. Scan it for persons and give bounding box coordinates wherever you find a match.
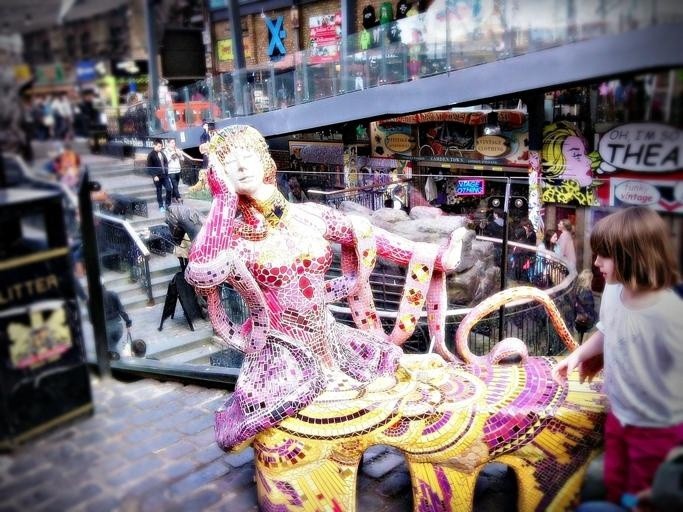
[550,201,682,505]
[541,120,602,207]
[382,184,597,325]
[183,123,460,456]
[19,85,191,366]
